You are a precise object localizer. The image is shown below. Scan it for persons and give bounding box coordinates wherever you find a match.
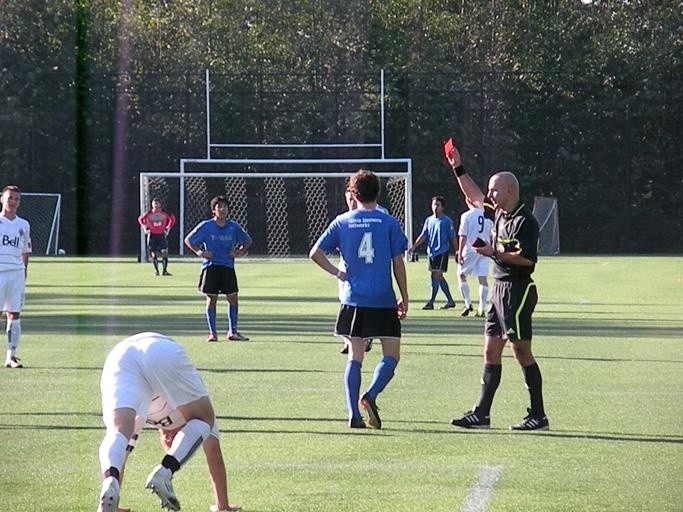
[409,196,459,309]
[448,147,550,431]
[309,171,408,429]
[0,186,33,369]
[138,198,176,275]
[335,186,372,353]
[97,332,243,512]
[184,196,252,342]
[374,203,389,215]
[458,198,494,317]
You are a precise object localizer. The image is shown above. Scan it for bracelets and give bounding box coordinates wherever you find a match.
[334,270,341,278]
[196,249,202,256]
[453,165,466,177]
[455,249,460,256]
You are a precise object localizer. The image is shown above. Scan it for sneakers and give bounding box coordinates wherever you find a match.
[340,344,348,353]
[360,392,381,429]
[473,311,484,317]
[451,409,490,429]
[207,332,217,341]
[349,417,365,428]
[146,468,181,512]
[227,332,249,341]
[461,305,473,316]
[98,476,121,512]
[162,271,172,275]
[422,303,433,310]
[5,356,23,368]
[439,301,455,310]
[509,415,549,431]
[365,338,372,352]
